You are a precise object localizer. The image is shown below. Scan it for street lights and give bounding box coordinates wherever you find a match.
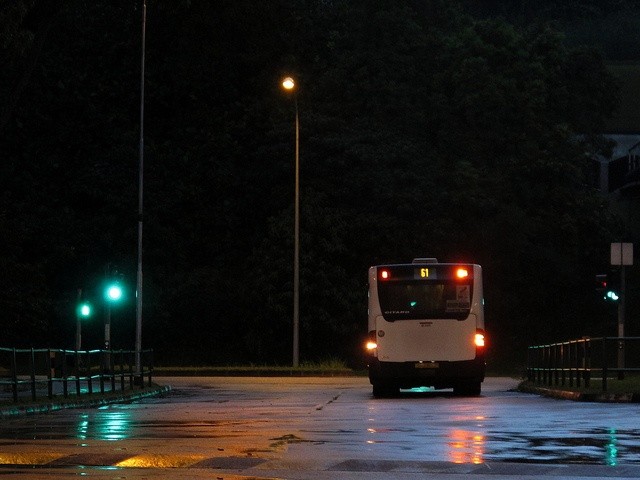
[105,285,120,381]
[281,77,300,371]
[75,300,91,378]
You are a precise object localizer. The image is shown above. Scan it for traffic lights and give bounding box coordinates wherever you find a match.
[595,272,608,300]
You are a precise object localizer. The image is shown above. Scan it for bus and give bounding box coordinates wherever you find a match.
[367,258,485,397]
